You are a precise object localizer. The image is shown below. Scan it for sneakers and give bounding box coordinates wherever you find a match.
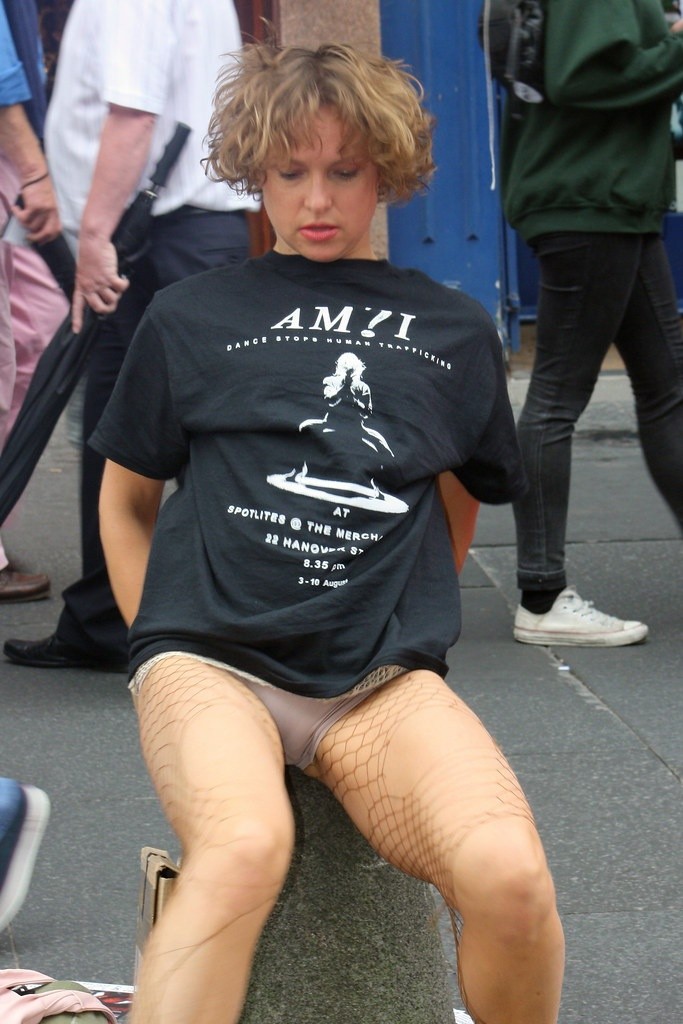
[514,586,650,647]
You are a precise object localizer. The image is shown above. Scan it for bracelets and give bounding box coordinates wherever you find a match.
[20,171,49,189]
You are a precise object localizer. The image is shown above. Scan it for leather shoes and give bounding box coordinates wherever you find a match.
[4,632,129,672]
[0,569,51,602]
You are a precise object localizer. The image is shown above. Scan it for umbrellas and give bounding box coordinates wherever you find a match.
[0,123,191,526]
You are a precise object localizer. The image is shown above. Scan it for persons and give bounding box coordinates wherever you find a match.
[0,776,50,935]
[500,0,683,646]
[98,42,565,1024]
[0,0,278,674]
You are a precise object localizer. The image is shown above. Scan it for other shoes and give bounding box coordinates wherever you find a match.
[0,778,52,932]
